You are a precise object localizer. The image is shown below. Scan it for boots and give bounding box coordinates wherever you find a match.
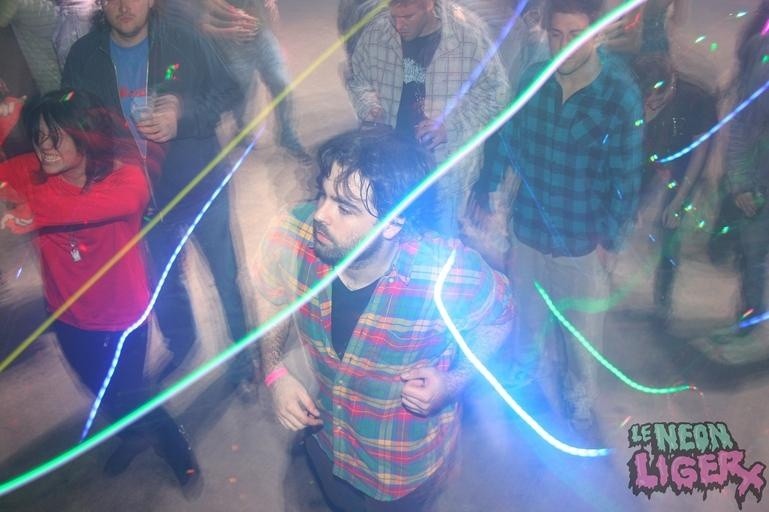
[105,432,151,476]
[166,434,201,491]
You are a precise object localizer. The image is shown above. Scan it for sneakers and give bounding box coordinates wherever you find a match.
[161,337,200,386]
[278,136,312,163]
[234,137,259,150]
[231,369,258,404]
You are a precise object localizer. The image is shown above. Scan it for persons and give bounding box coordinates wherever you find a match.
[0,0,769,512]
